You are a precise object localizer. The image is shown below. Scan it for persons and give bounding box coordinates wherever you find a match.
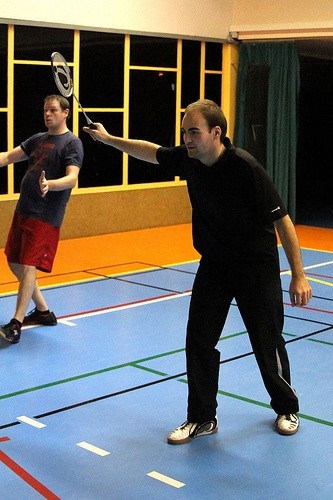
[80,99,313,444]
[0,95,84,344]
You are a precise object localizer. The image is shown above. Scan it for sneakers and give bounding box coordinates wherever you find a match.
[0,319,21,343]
[166,415,217,444]
[21,309,56,325]
[274,413,299,436]
